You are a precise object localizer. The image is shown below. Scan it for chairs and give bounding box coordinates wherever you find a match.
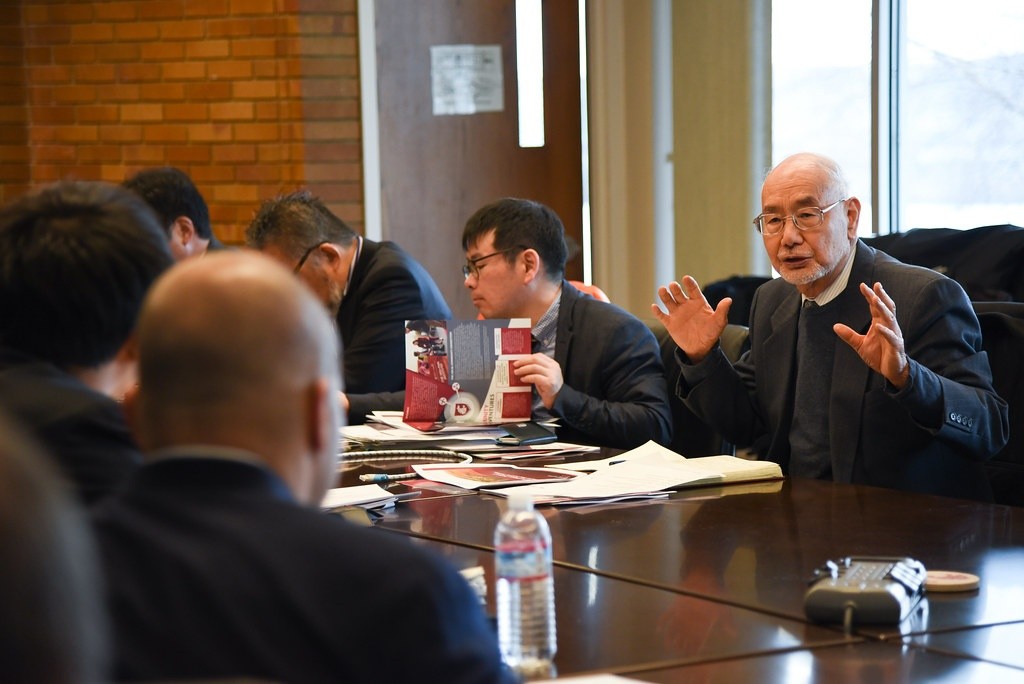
[861,224,1024,507]
[651,274,773,460]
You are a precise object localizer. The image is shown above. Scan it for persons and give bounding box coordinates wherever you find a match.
[651,152,1008,496]
[85,250,518,684]
[337,198,670,461]
[124,167,224,256]
[405,320,447,358]
[656,484,997,684]
[249,192,451,393]
[0,182,172,502]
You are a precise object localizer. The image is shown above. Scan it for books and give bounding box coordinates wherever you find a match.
[678,456,785,489]
[320,484,395,507]
[342,449,455,457]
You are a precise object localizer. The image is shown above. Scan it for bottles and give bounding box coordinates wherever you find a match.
[494,488,558,667]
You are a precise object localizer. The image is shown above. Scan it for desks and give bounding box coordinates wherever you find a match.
[317,429,1024,684]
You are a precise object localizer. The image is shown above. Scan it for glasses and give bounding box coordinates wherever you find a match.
[753,199,844,236]
[462,245,529,280]
[290,239,332,272]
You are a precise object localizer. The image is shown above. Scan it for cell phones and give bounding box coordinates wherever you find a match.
[500,422,558,445]
